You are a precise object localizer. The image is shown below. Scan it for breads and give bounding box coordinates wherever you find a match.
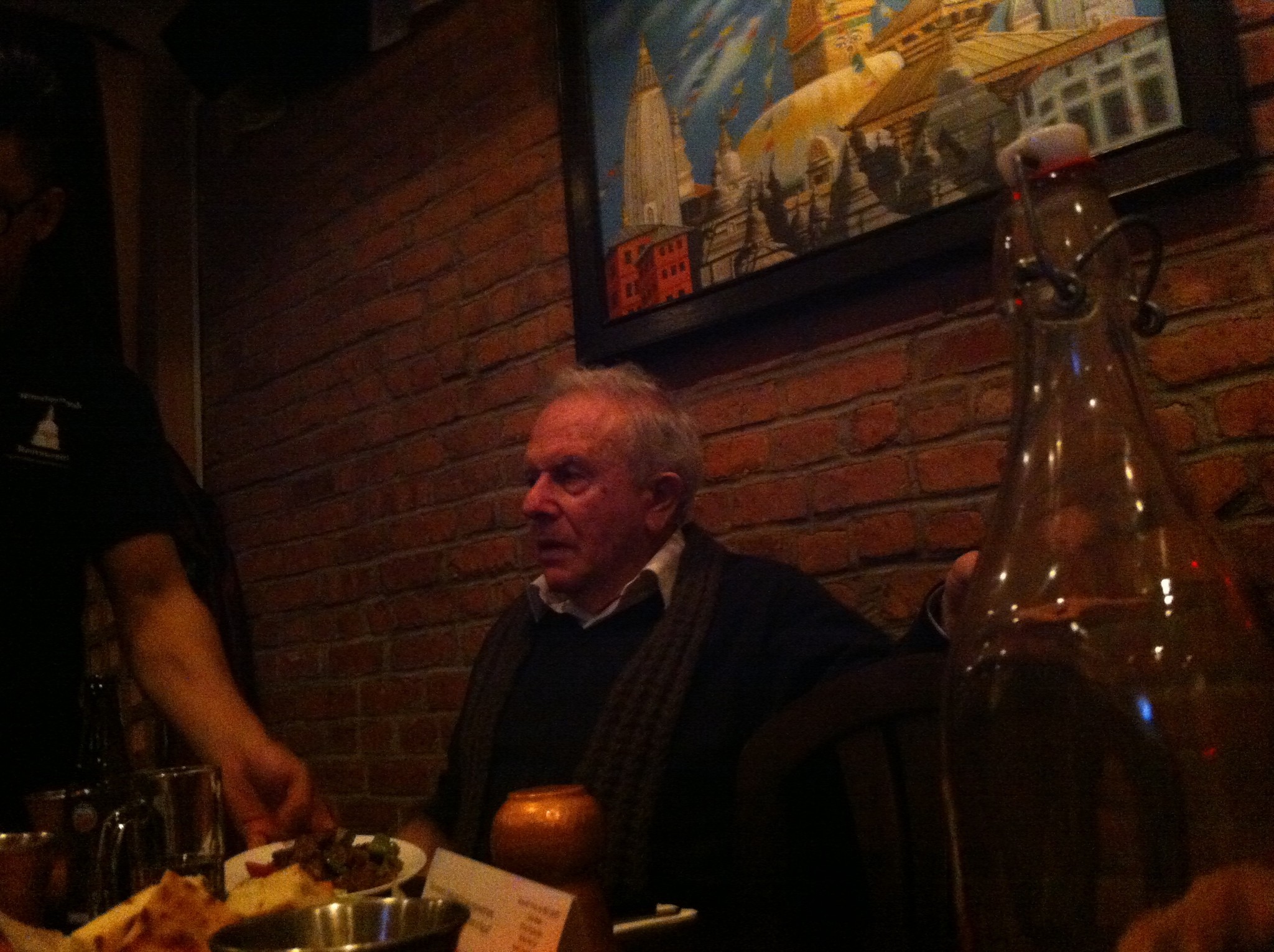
[0,864,334,951]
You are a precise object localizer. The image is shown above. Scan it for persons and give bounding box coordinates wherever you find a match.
[0,70,336,853]
[388,361,987,952]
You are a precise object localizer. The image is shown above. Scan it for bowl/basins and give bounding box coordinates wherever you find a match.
[206,896,470,952]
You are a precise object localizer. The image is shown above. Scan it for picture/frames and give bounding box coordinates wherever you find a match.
[539,0,1254,378]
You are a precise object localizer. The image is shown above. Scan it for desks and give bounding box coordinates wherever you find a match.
[0,796,622,952]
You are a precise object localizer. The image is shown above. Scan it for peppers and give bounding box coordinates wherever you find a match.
[273,826,405,877]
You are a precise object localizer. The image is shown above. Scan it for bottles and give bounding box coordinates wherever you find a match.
[494,783,611,952]
[940,121,1274,952]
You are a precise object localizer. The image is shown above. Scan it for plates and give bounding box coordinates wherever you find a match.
[221,834,432,898]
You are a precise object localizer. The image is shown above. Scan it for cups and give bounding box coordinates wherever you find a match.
[98,761,221,911]
[28,788,98,913]
[1,831,50,925]
